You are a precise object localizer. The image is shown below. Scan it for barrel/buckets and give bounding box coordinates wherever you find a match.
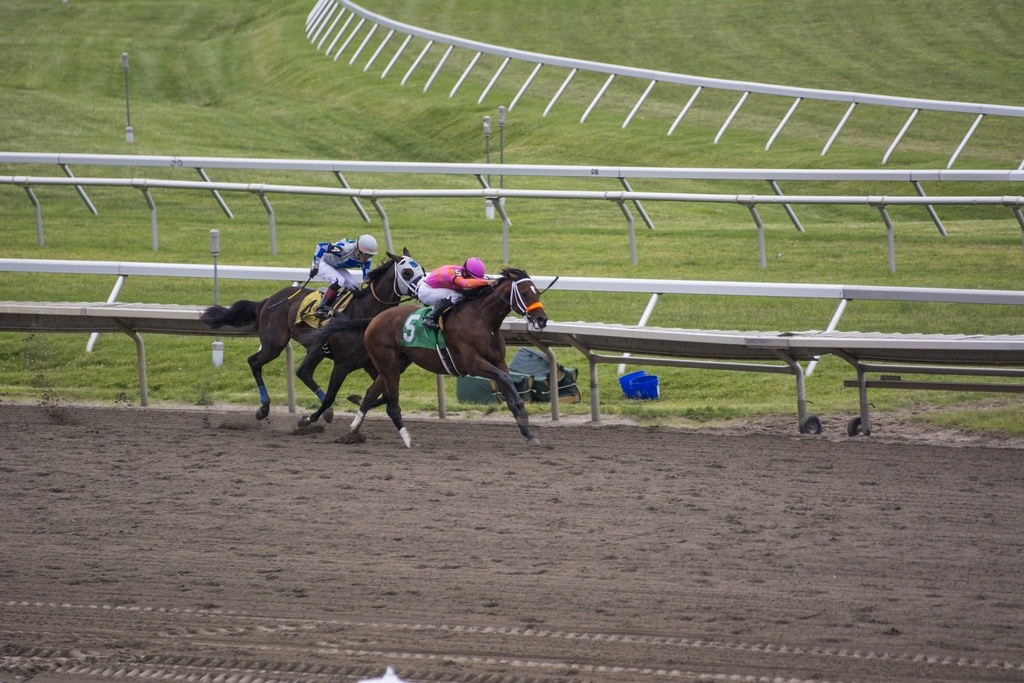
[619,370,648,398]
[630,375,660,400]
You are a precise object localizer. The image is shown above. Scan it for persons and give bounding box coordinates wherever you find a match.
[418,257,498,331]
[309,234,379,318]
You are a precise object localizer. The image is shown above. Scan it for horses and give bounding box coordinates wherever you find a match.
[198,248,431,429]
[345,267,549,448]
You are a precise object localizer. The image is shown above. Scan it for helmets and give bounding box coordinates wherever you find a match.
[462,257,485,279]
[356,234,377,255]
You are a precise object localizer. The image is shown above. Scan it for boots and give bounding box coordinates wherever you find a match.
[423,298,454,329]
[316,283,341,318]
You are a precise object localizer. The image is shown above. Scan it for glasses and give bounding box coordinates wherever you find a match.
[361,254,370,258]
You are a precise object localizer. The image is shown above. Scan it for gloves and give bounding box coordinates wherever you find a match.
[309,268,318,278]
